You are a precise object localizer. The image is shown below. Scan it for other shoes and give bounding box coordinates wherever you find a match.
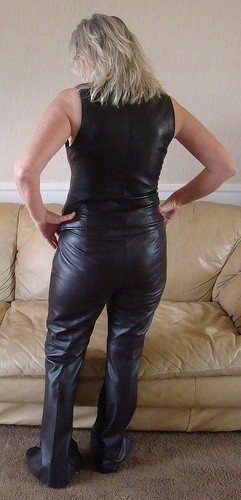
[26,446,40,460]
[98,463,117,474]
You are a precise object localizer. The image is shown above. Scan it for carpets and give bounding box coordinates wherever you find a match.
[0,422,240,500]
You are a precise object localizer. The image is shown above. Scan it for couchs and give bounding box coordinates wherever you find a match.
[0,201,241,431]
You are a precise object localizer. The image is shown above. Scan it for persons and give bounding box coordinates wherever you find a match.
[10,13,238,488]
